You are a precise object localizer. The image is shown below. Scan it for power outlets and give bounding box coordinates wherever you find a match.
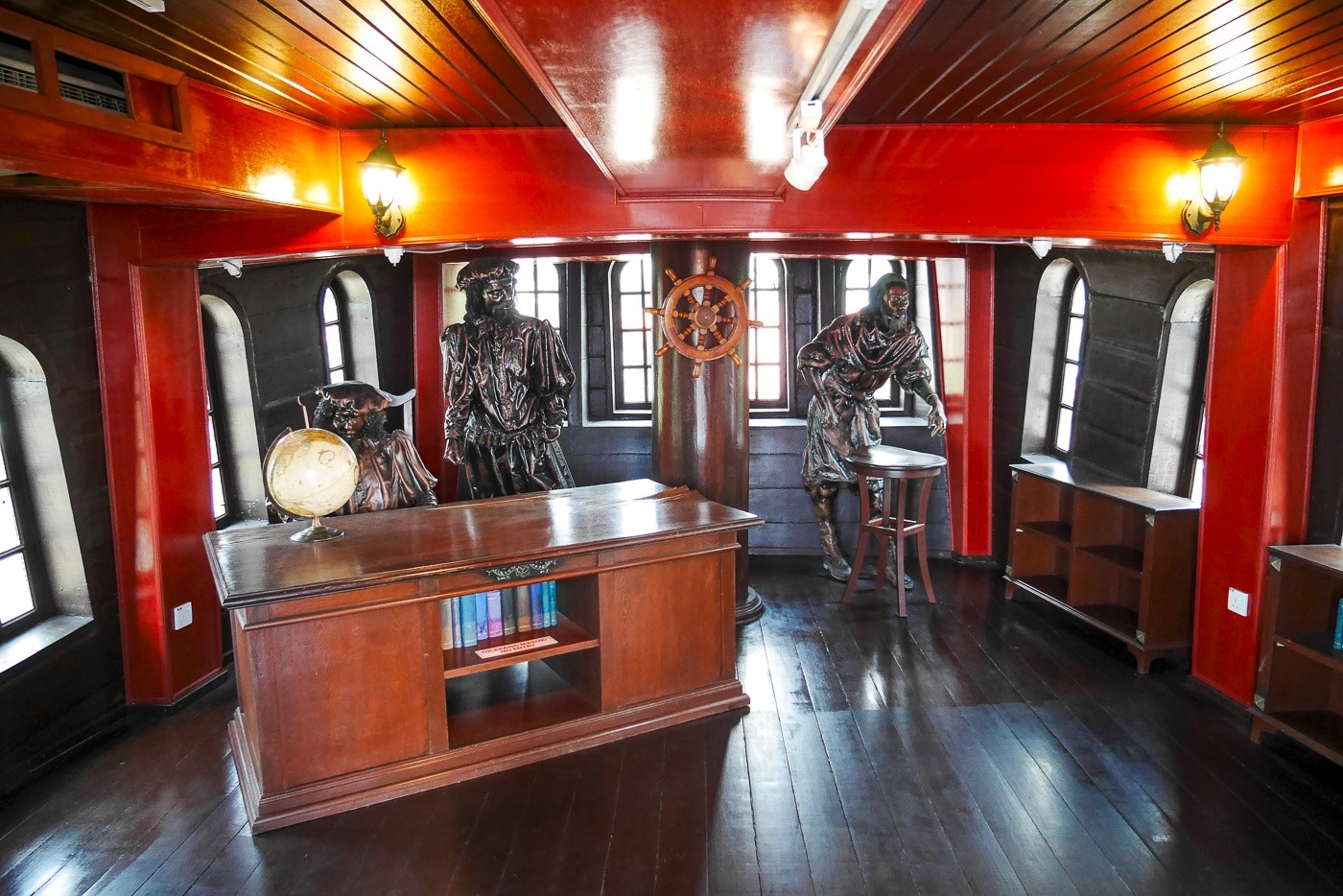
[1228,587,1249,617]
[173,601,193,631]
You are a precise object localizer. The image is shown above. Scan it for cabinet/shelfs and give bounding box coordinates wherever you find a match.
[1249,542,1343,768]
[1001,460,1201,676]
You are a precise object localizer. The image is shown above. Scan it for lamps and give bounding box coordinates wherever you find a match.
[1180,120,1250,236]
[354,125,408,239]
[782,100,828,191]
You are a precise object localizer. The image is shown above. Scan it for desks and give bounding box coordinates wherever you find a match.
[840,445,948,617]
[201,477,768,841]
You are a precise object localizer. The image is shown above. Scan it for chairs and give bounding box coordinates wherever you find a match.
[296,380,417,441]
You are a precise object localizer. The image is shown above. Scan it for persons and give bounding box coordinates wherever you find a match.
[313,383,439,517]
[439,255,577,499]
[796,272,947,590]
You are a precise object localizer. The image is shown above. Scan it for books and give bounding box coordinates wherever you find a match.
[438,580,557,651]
